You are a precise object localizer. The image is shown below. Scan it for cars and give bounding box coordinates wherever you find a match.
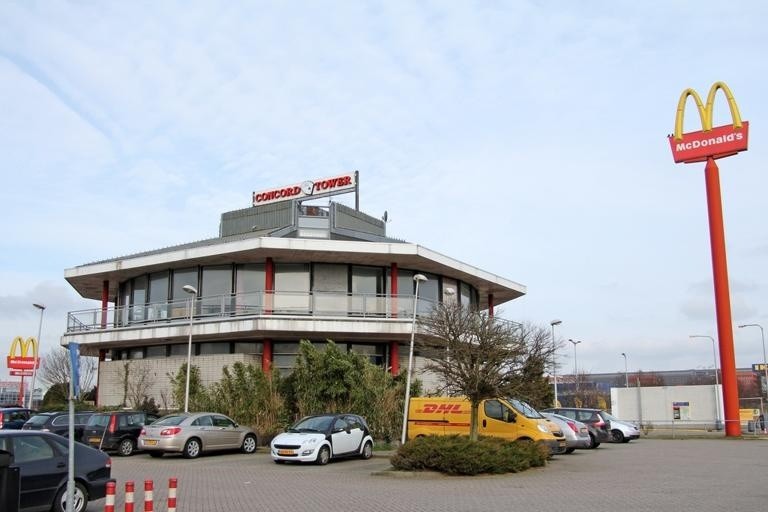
[267,413,375,468]
[0,404,159,512]
[136,411,261,460]
[528,405,642,454]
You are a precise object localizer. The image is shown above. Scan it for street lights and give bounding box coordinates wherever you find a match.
[397,270,430,448]
[442,287,457,398]
[687,334,722,431]
[619,351,629,387]
[27,302,47,411]
[735,323,767,400]
[181,283,198,412]
[567,337,582,393]
[549,318,562,407]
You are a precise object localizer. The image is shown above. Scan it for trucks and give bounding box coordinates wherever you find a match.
[406,395,566,459]
